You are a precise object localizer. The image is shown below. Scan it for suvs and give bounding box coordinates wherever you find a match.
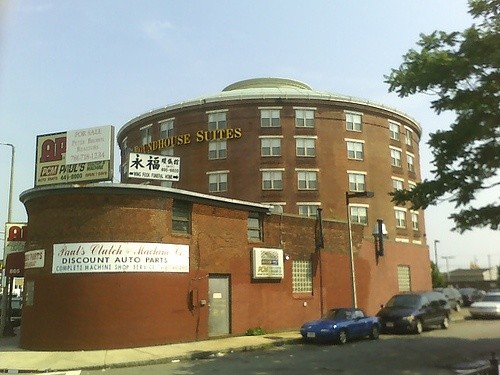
[433,288,463,312]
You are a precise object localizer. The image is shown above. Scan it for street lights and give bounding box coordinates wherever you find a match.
[345,191,375,308]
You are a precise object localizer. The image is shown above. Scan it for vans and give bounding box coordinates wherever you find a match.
[375,290,452,335]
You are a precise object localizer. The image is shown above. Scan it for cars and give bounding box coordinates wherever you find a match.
[469,292,500,318]
[300,307,381,345]
[459,288,479,305]
